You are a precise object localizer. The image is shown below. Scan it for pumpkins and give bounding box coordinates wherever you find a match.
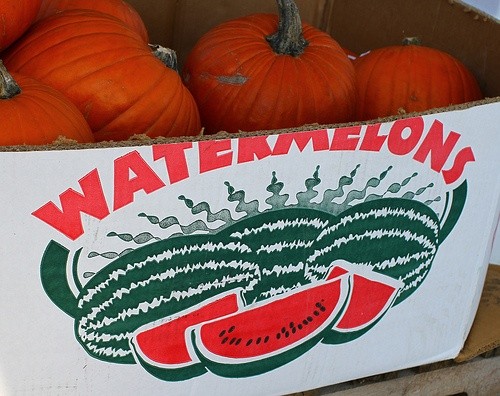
[0,1,482,147]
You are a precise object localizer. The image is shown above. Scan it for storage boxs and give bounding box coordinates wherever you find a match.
[0,0,500,396]
